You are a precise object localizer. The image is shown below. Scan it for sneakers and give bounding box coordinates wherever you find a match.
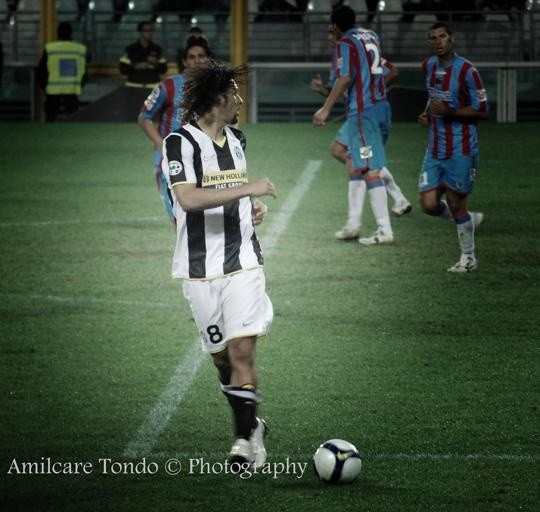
[250,416,269,470]
[391,198,411,217]
[446,255,478,272]
[228,438,254,470]
[334,224,359,240]
[467,210,485,225]
[358,231,395,245]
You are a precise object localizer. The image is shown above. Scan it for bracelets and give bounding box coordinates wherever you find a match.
[444,106,456,117]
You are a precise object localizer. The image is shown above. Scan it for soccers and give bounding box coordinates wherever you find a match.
[315,438,363,483]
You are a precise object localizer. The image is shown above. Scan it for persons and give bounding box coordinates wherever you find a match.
[34,21,93,124]
[310,3,397,247]
[175,26,205,72]
[118,20,168,121]
[309,20,414,218]
[418,23,491,275]
[79,15,499,96]
[137,35,216,234]
[160,60,280,474]
